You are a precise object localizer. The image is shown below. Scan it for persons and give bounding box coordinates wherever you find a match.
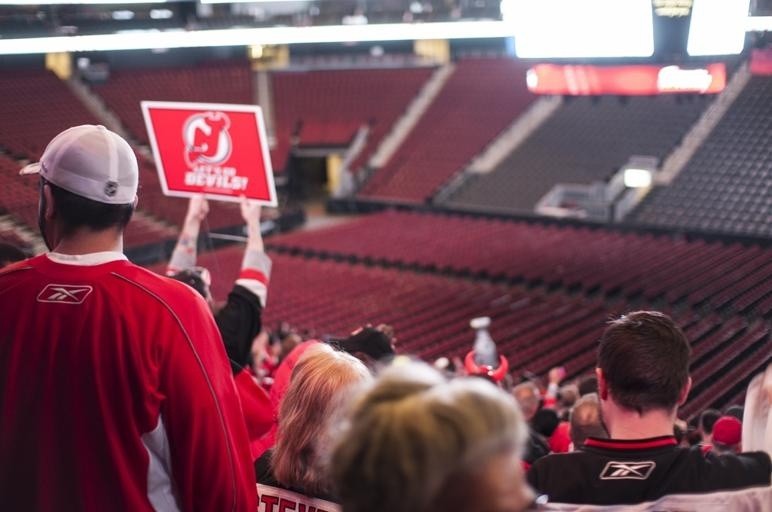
[524,311,772,512]
[0,124,258,512]
[162,193,537,512]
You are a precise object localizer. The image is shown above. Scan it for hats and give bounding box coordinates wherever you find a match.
[331,329,393,359]
[165,265,213,298]
[16,123,140,204]
[712,415,742,447]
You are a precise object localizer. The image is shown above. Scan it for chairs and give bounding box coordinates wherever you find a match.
[0,58,772,423]
